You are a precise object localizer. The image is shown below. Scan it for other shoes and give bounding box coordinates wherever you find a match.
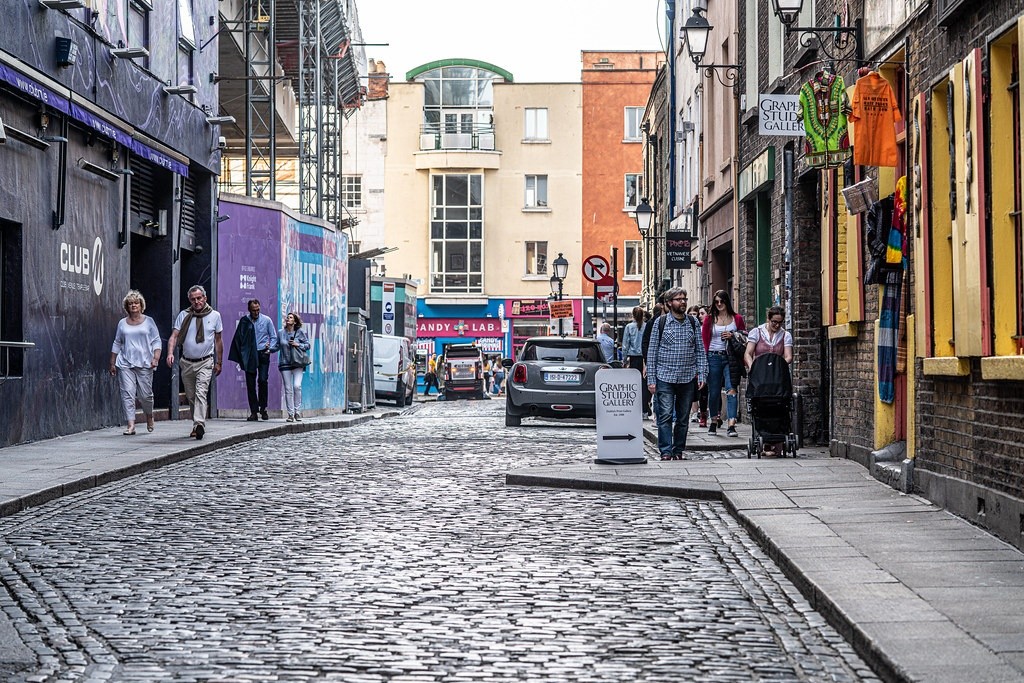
[661,455,682,460]
[498,390,502,397]
[123,426,136,435]
[762,451,777,457]
[691,411,699,423]
[651,423,658,428]
[642,412,649,420]
[259,410,269,420]
[717,419,723,428]
[699,412,708,427]
[726,425,738,437]
[708,423,718,436]
[247,413,258,421]
[190,425,205,440]
[286,414,302,422]
[147,414,154,432]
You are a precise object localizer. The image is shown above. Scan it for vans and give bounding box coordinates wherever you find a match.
[372,334,416,407]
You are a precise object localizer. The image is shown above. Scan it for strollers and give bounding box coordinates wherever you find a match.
[744,353,796,459]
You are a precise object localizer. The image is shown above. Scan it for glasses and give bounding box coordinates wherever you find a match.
[715,299,725,304]
[673,298,688,303]
[771,320,785,324]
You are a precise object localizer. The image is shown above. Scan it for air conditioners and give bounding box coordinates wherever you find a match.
[682,121,694,134]
[675,131,684,141]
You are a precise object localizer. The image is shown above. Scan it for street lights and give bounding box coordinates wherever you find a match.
[550,272,559,301]
[552,253,570,336]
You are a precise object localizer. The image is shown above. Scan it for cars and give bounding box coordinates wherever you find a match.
[501,336,613,427]
[442,343,489,400]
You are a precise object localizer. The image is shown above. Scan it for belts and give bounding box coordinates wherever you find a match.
[708,351,727,355]
[182,354,213,362]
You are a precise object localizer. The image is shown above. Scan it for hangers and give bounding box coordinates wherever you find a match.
[804,50,894,84]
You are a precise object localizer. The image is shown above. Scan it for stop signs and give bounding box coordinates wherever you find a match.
[597,276,619,304]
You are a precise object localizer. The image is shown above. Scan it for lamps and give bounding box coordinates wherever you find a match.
[163,85,198,95]
[680,7,741,87]
[772,0,864,68]
[205,116,236,125]
[110,47,151,58]
[38,0,86,10]
[633,198,699,253]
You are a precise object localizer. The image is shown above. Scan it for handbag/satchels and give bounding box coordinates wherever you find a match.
[290,329,312,368]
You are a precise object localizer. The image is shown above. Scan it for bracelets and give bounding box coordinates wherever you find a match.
[217,361,222,366]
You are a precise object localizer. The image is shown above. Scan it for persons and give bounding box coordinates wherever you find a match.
[647,287,707,461]
[265,312,311,422]
[109,289,162,435]
[702,289,745,437]
[424,352,442,396]
[597,303,725,428]
[744,306,793,456]
[166,285,223,439]
[483,355,506,397]
[241,299,277,421]
